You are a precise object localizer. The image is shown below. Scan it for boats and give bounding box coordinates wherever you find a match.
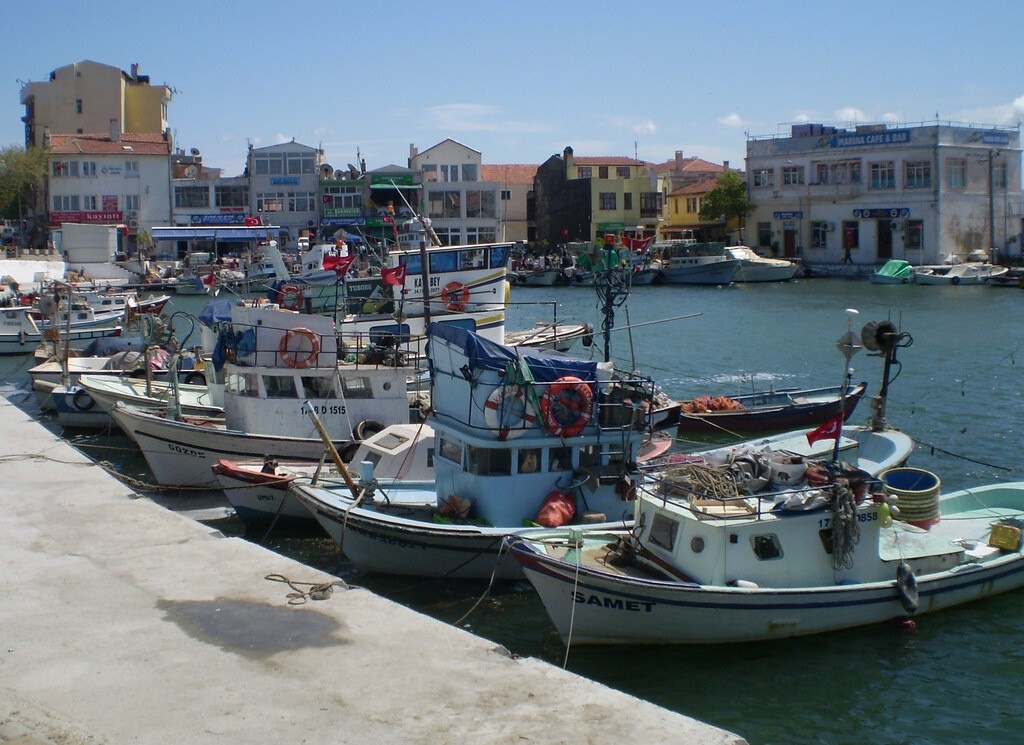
[866,259,913,285]
[285,234,914,590]
[210,417,674,537]
[910,263,1007,286]
[0,211,807,496]
[985,266,1024,287]
[497,307,1023,647]
[667,380,867,433]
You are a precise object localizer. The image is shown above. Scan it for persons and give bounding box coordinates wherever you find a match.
[206,249,240,270]
[462,249,485,268]
[0,274,116,310]
[509,245,574,270]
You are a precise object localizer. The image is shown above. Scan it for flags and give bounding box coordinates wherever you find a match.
[381,264,405,285]
[323,254,354,276]
[805,414,842,448]
[245,216,263,226]
[204,273,215,286]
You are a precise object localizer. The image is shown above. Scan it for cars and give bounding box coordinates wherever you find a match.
[966,249,989,263]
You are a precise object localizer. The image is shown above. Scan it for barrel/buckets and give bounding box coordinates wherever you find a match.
[877,466,942,531]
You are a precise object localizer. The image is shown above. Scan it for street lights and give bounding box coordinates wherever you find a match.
[503,166,511,237]
[787,159,801,247]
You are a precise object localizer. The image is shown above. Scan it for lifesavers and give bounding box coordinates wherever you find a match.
[185,371,207,385]
[442,281,469,310]
[279,327,320,368]
[805,466,869,507]
[485,383,536,438]
[130,369,158,381]
[338,441,360,463]
[541,375,593,436]
[353,419,386,441]
[582,322,594,346]
[72,389,95,412]
[952,276,960,285]
[903,278,909,283]
[276,286,303,311]
